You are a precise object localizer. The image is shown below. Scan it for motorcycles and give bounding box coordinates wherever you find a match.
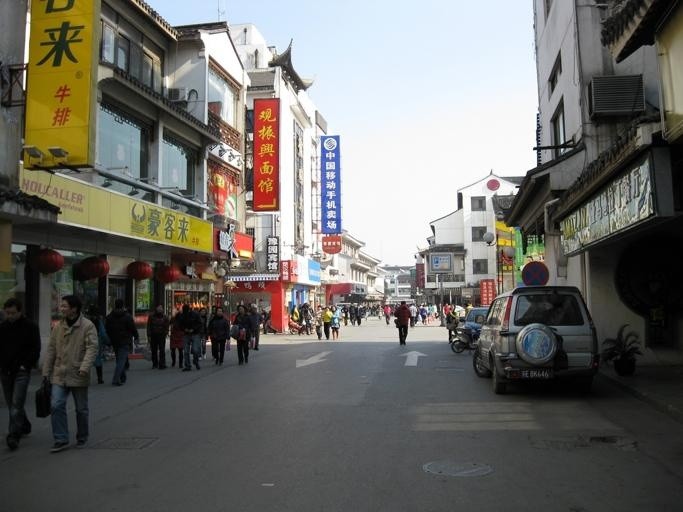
[288,319,316,335]
[446,327,482,354]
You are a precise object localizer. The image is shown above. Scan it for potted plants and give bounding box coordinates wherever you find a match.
[600,323,646,374]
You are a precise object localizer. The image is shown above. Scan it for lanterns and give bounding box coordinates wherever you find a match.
[79,256,110,278]
[156,264,180,283]
[29,248,65,278]
[126,261,152,280]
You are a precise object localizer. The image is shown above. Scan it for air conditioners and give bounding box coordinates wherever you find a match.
[166,86,191,101]
[588,71,646,117]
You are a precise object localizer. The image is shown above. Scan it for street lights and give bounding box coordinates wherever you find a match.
[503,248,516,289]
[483,231,500,297]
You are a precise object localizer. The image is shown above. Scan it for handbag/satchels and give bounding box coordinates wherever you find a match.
[35,384,52,418]
[144,347,152,361]
[230,325,246,340]
[226,342,230,351]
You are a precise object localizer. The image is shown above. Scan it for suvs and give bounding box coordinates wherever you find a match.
[472,285,599,394]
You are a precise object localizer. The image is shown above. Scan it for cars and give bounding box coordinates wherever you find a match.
[336,302,366,320]
[464,306,490,342]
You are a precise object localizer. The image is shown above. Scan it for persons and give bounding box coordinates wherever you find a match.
[290,299,459,347]
[41,294,99,453]
[0,297,41,451]
[86,298,271,386]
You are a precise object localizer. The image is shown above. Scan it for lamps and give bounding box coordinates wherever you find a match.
[99,166,221,214]
[48,146,70,164]
[20,143,46,166]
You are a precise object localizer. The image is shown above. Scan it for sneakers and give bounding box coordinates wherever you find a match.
[76,439,88,448]
[49,440,70,452]
